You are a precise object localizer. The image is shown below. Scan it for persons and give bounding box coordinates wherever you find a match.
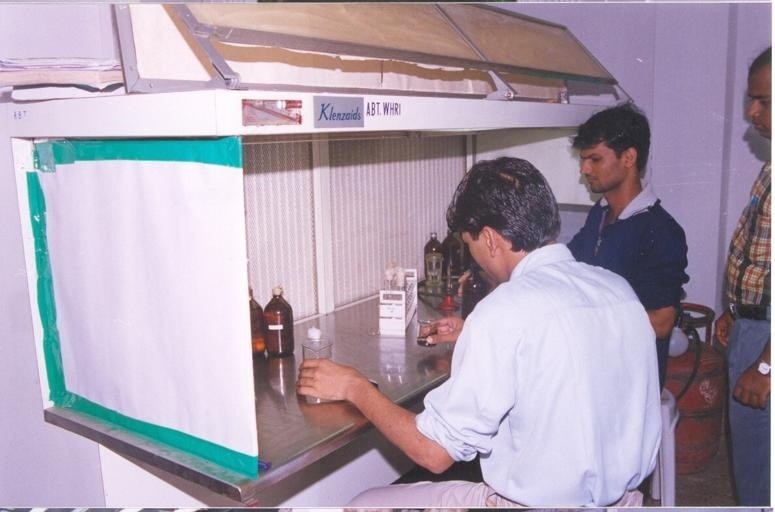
[456,100,690,394]
[714,45,771,505]
[294,156,663,508]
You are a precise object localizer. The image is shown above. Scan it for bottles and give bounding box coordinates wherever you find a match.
[380,262,408,299]
[424,230,466,278]
[462,263,488,320]
[249,288,294,358]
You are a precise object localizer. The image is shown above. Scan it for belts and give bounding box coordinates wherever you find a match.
[729,303,766,320]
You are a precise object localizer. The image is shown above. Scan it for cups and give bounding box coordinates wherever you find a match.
[425,254,443,284]
[300,338,333,402]
[417,319,438,348]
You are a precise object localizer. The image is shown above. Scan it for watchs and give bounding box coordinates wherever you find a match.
[755,358,770,377]
